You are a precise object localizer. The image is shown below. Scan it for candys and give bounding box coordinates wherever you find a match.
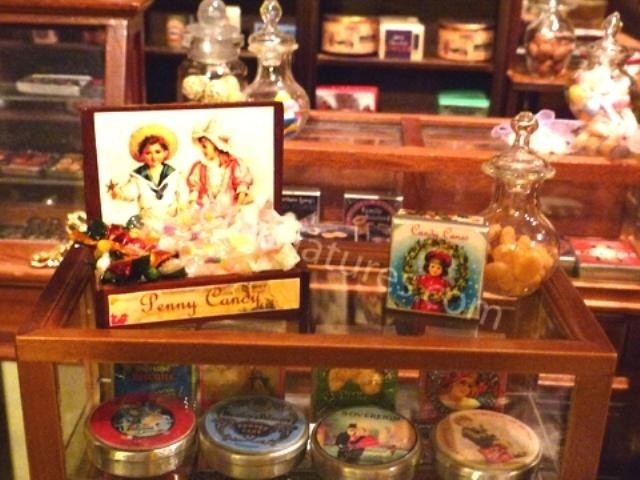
[480,225,552,298]
[525,30,576,77]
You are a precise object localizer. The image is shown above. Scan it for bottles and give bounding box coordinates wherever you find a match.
[463,108,563,308]
[235,0,316,146]
[523,0,575,78]
[561,9,636,130]
[174,0,249,104]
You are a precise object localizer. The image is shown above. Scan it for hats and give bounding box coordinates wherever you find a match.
[129,123,178,162]
[192,121,233,154]
[426,251,452,267]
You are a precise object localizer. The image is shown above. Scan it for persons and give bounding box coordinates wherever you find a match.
[334,423,357,459]
[349,427,378,449]
[413,252,454,313]
[103,121,182,215]
[187,120,256,213]
[451,414,512,463]
[380,420,407,457]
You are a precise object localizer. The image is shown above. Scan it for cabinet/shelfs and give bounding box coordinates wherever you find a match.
[0,1,640,479]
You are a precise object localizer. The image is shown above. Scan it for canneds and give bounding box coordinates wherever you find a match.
[437,19,496,63]
[320,13,377,56]
